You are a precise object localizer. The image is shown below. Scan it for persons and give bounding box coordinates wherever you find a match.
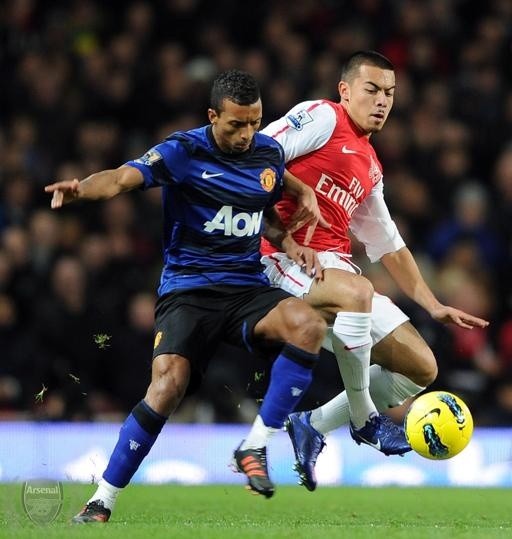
[253,51,493,494]
[0,1,512,426]
[42,69,330,526]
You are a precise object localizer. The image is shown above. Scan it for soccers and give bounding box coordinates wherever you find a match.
[403,390,474,459]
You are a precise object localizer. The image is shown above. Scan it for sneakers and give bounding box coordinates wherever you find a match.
[286,411,324,491]
[226,441,275,497]
[350,414,411,455]
[71,499,112,525]
